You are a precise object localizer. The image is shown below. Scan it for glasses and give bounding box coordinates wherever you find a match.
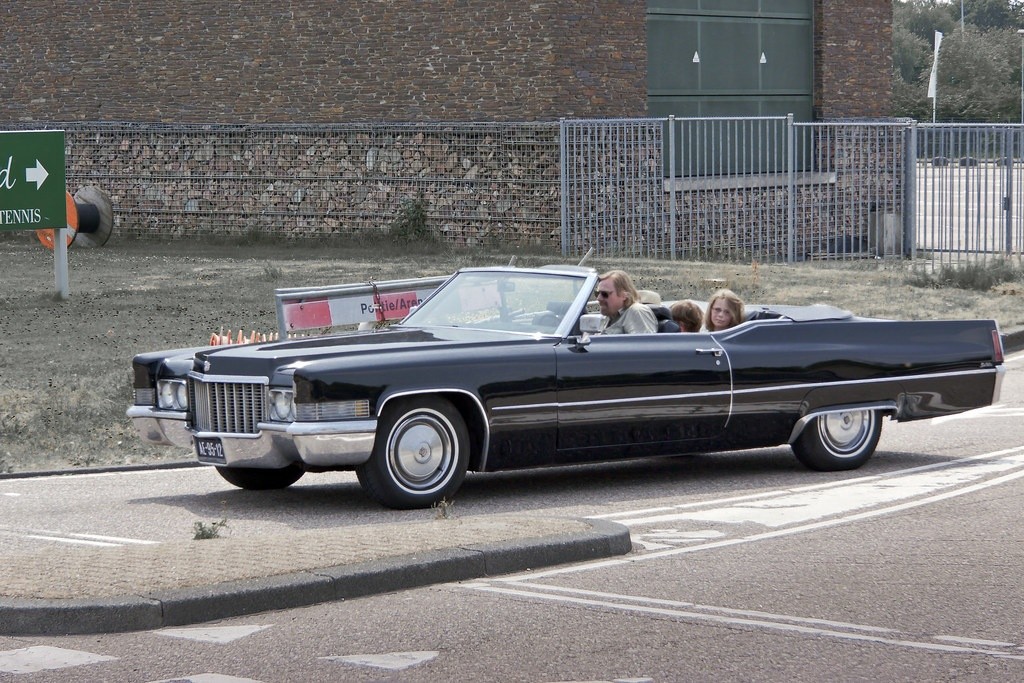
[712,307,731,315]
[594,291,613,299]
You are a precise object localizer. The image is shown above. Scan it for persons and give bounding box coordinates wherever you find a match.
[594,269,659,335]
[706,287,748,332]
[670,300,707,333]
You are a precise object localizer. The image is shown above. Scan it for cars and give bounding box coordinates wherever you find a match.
[125,246,1007,509]
